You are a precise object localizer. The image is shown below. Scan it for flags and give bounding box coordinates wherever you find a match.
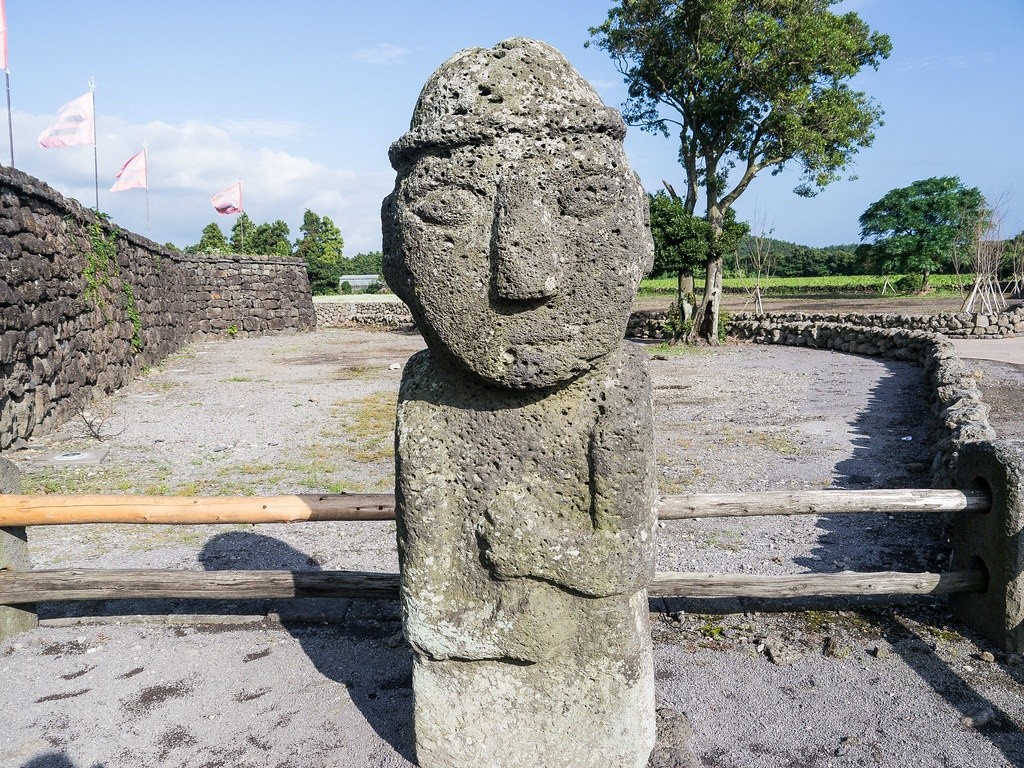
[108,149,148,190]
[209,180,242,215]
[37,91,97,151]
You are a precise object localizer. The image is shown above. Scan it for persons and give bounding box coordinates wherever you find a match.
[380,35,659,768]
